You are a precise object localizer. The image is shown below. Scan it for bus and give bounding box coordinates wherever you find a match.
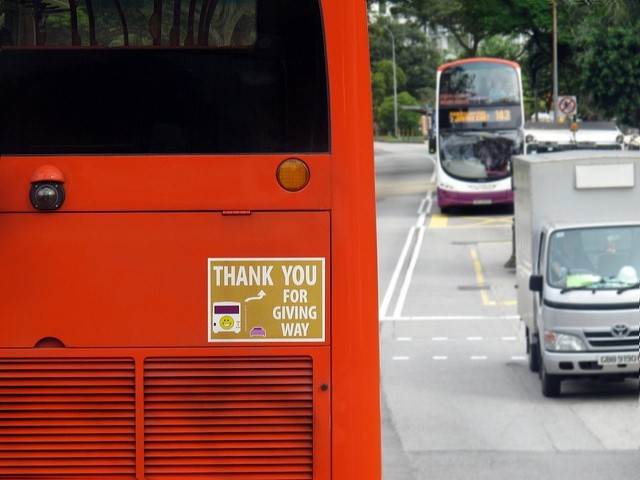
[0,0,381,480]
[428,57,525,213]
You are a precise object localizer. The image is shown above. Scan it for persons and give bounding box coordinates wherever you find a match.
[551,233,594,278]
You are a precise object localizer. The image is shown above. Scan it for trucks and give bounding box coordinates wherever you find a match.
[512,149,640,397]
